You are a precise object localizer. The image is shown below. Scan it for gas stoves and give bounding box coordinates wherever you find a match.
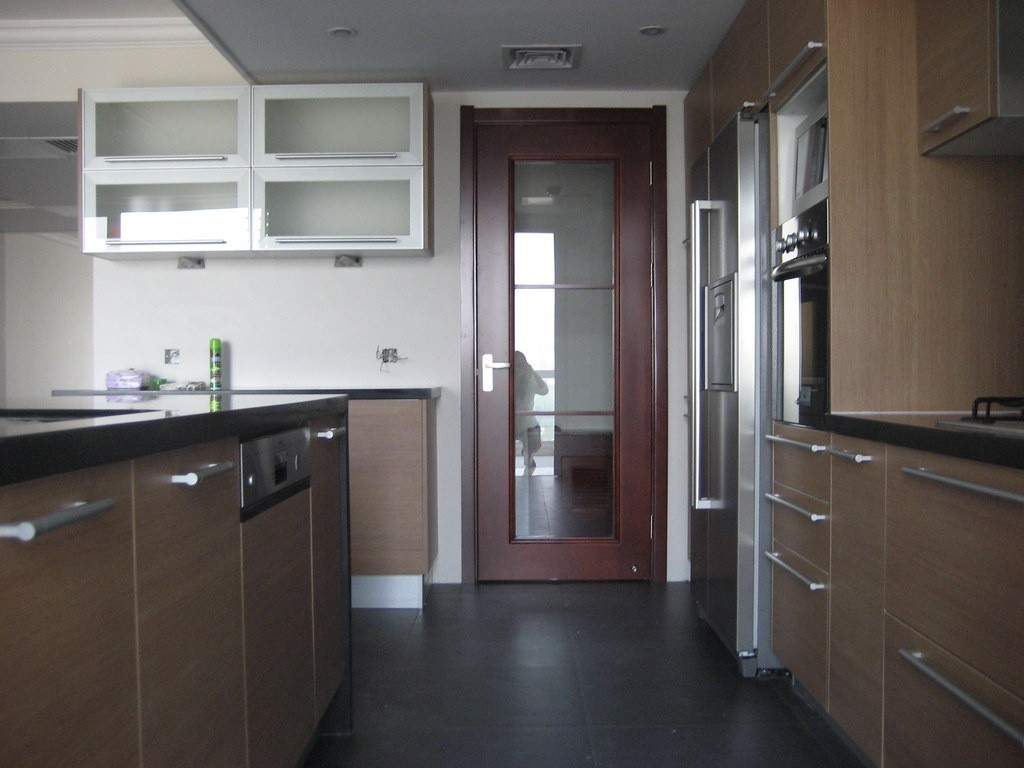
[936,397,1024,433]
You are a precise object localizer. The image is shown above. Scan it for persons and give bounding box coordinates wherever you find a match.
[514,351,549,476]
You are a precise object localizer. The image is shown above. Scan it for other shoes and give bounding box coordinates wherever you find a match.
[522,450,537,467]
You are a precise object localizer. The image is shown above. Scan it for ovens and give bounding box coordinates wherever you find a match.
[771,198,829,430]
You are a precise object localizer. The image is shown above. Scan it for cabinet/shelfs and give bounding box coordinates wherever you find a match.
[347,399,437,609]
[0,461,142,768]
[241,488,315,768]
[77,82,433,256]
[308,412,351,740]
[764,419,1023,768]
[684,0,1024,171]
[131,436,248,768]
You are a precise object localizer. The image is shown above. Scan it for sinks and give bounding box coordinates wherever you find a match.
[0,409,164,423]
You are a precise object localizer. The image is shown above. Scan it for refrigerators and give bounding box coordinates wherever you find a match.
[686,109,786,678]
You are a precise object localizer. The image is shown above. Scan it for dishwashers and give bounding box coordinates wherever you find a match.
[242,426,314,768]
